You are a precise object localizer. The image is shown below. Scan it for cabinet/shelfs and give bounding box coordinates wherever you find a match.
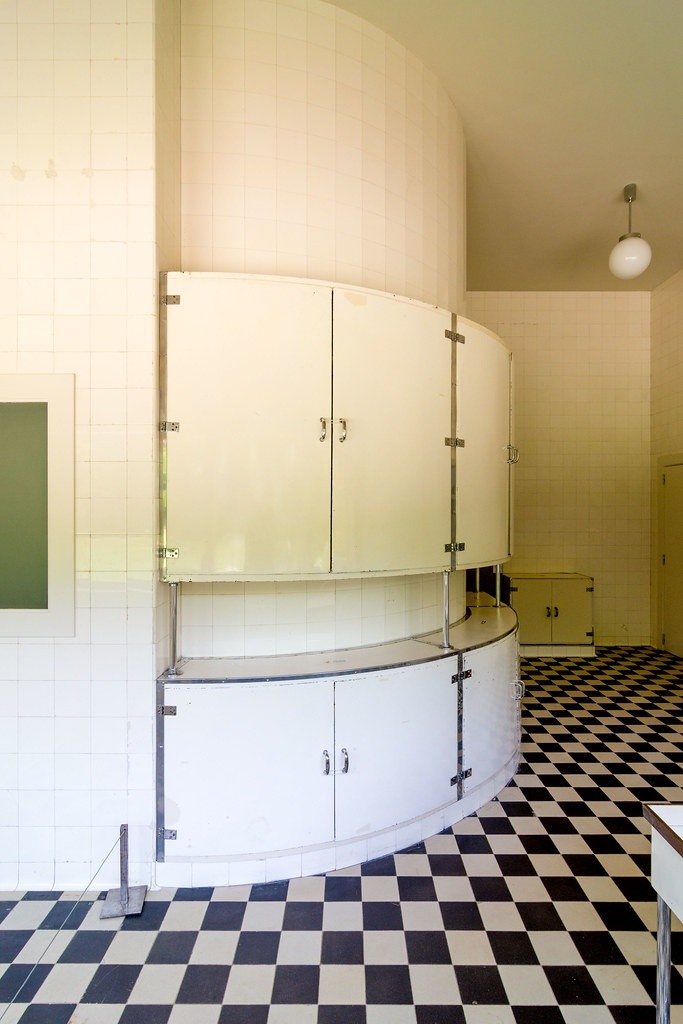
[158,593,519,884]
[507,570,595,653]
[159,272,513,581]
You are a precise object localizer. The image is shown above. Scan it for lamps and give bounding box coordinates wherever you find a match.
[607,186,653,277]
